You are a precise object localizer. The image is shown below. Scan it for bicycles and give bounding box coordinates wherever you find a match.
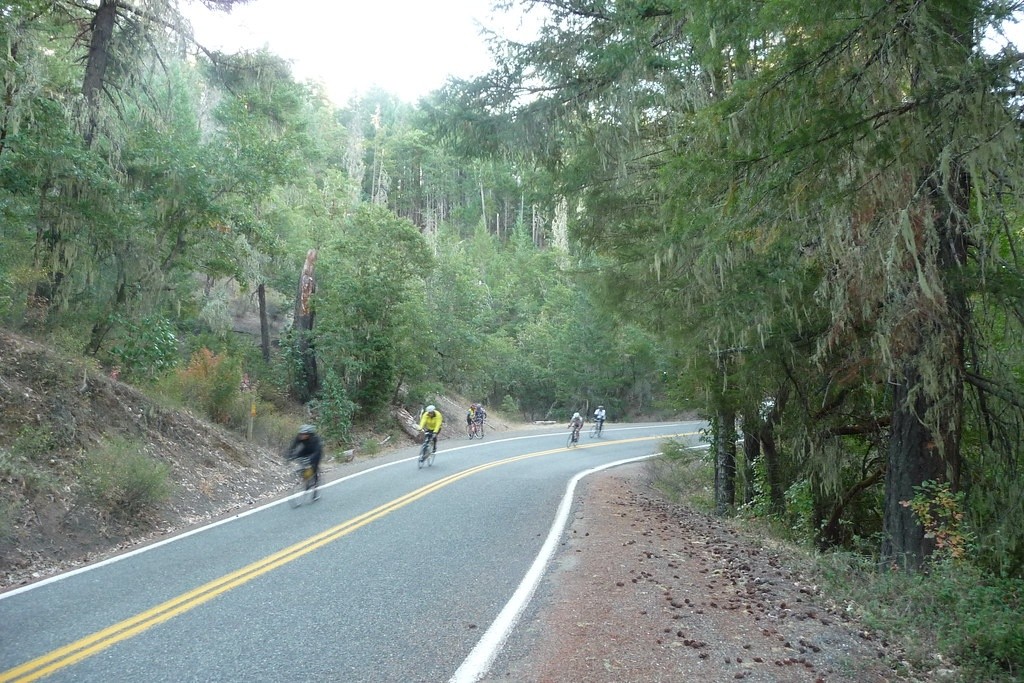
[590,419,605,439]
[416,427,437,470]
[567,423,579,448]
[287,454,324,508]
[466,416,484,439]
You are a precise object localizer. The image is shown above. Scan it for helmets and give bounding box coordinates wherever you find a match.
[470,404,476,409]
[477,404,481,409]
[598,404,604,407]
[298,425,317,433]
[574,413,579,418]
[425,405,435,412]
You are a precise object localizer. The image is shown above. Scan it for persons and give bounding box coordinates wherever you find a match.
[285,424,322,499]
[418,404,443,462]
[592,405,606,430]
[467,403,486,437]
[569,412,583,441]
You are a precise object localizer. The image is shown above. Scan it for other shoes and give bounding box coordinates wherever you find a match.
[433,448,436,453]
